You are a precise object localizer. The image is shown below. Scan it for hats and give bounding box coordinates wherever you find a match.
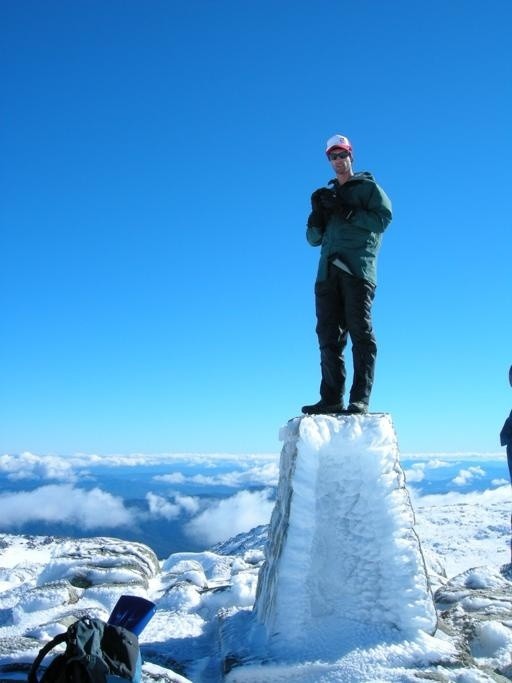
[326,135,352,155]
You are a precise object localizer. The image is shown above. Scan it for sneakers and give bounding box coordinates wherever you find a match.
[347,401,368,414]
[301,401,345,414]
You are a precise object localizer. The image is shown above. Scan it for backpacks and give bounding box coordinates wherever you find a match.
[26,615,142,683]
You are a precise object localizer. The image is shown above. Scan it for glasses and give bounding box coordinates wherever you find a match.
[328,150,349,161]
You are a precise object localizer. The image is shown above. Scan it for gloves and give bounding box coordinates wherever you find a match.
[308,187,356,227]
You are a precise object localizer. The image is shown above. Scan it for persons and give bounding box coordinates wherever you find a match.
[301,133,393,414]
